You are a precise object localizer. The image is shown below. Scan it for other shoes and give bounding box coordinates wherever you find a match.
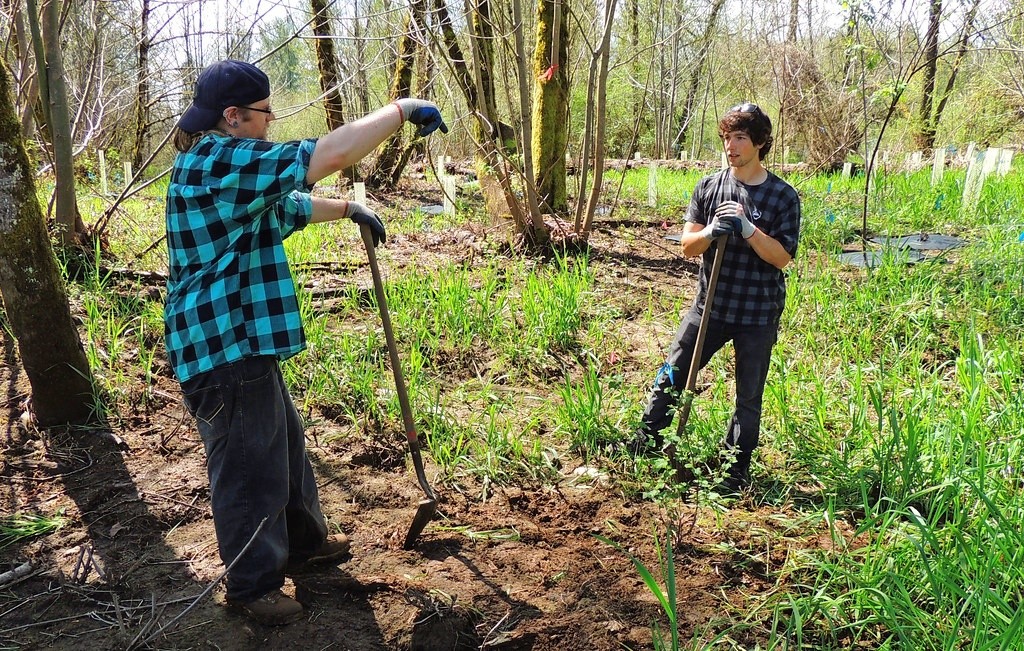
[709,467,753,495]
[227,583,303,625]
[600,430,663,456]
[296,533,350,565]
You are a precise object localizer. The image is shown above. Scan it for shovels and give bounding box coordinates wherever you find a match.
[359,218,440,547]
[665,229,728,462]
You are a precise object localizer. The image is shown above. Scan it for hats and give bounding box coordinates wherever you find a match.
[176,60,270,133]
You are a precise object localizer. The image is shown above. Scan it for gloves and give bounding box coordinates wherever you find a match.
[703,214,739,241]
[715,201,756,239]
[393,98,449,137]
[343,201,386,247]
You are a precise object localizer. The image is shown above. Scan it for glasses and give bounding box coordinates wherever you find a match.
[729,103,763,123]
[234,106,272,116]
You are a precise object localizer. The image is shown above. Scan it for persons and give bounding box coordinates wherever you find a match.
[162,60,449,624]
[624,103,800,494]
